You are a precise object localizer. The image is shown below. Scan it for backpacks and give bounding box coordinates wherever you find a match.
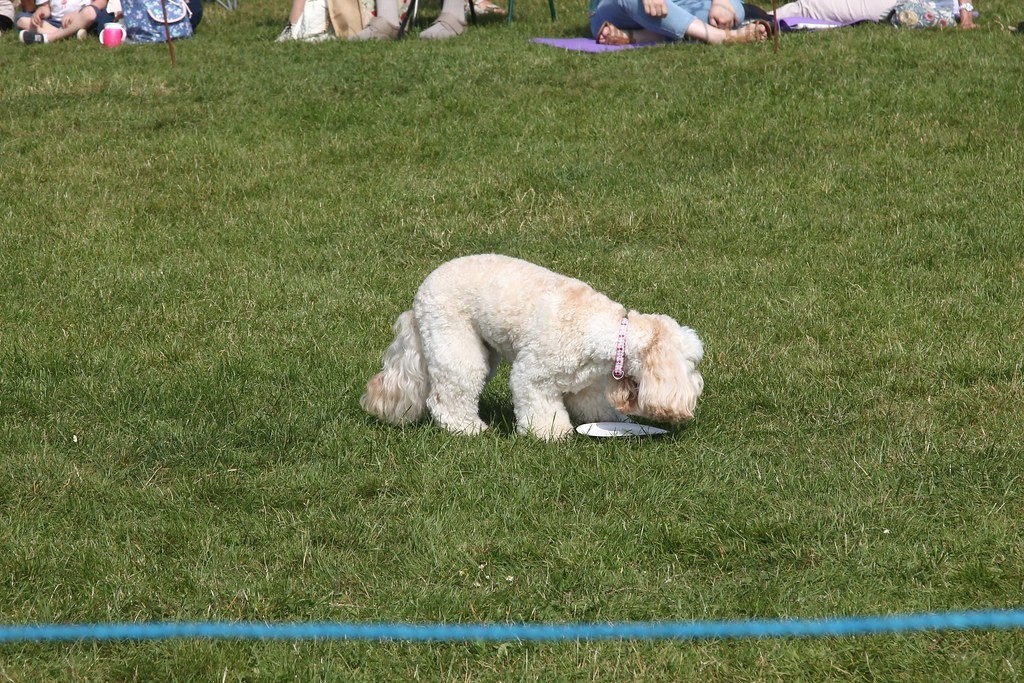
[120,0,192,45]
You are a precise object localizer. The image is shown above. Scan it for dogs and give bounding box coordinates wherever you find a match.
[358,254,705,441]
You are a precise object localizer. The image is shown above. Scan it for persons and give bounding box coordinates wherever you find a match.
[0,0,202,44]
[767,0,979,29]
[588,0,771,46]
[275,0,510,44]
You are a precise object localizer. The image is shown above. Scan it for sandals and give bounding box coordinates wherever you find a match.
[596,20,636,45]
[724,19,772,45]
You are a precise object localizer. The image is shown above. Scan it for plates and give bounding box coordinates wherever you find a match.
[575,422,671,443]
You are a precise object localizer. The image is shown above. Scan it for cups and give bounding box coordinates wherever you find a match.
[99,23,126,48]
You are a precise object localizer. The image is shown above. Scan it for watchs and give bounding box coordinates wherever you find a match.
[959,3,974,11]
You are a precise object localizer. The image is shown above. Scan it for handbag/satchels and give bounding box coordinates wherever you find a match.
[880,0,957,28]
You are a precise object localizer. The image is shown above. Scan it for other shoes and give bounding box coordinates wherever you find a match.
[19,29,44,45]
[347,14,401,41]
[420,13,468,39]
[274,23,299,43]
[466,0,508,16]
[72,29,87,41]
